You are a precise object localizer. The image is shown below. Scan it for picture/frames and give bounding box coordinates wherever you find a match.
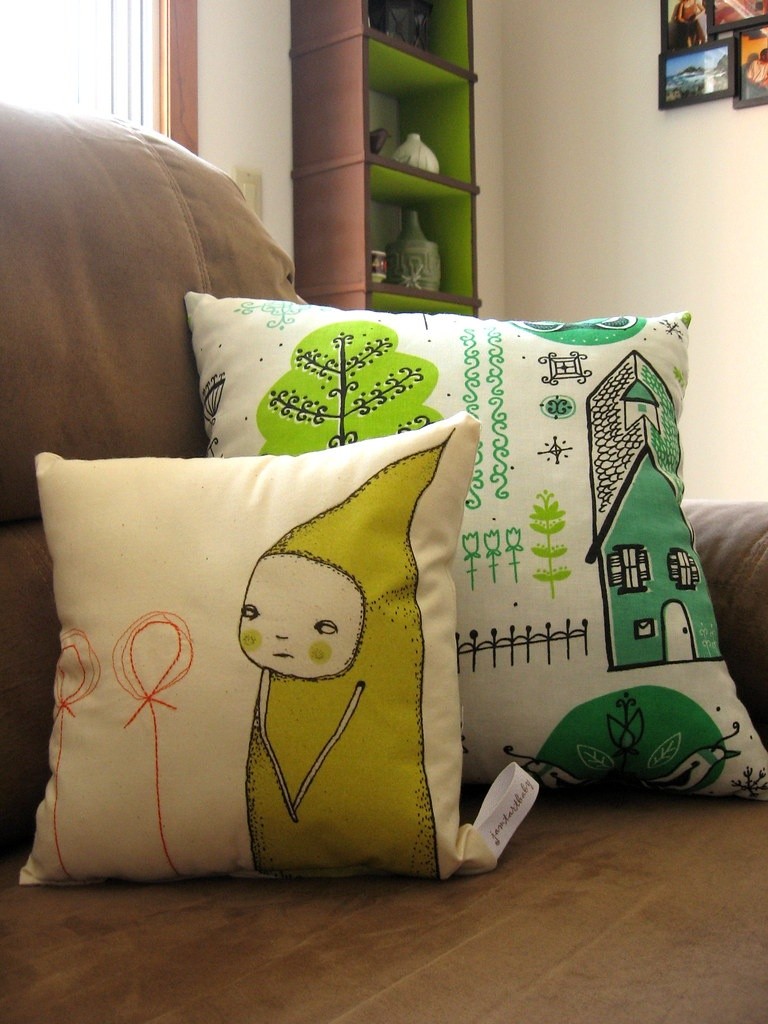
[707,0,768,35]
[732,22,768,109]
[658,36,738,110]
[660,0,717,55]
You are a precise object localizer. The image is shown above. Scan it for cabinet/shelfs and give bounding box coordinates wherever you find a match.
[290,0,481,319]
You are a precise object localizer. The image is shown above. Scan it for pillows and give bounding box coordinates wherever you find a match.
[184,291,768,799]
[17,410,538,886]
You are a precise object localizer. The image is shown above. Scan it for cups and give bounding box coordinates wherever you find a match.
[371,251,387,283]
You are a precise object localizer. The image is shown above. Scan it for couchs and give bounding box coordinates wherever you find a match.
[1,102,768,1024]
